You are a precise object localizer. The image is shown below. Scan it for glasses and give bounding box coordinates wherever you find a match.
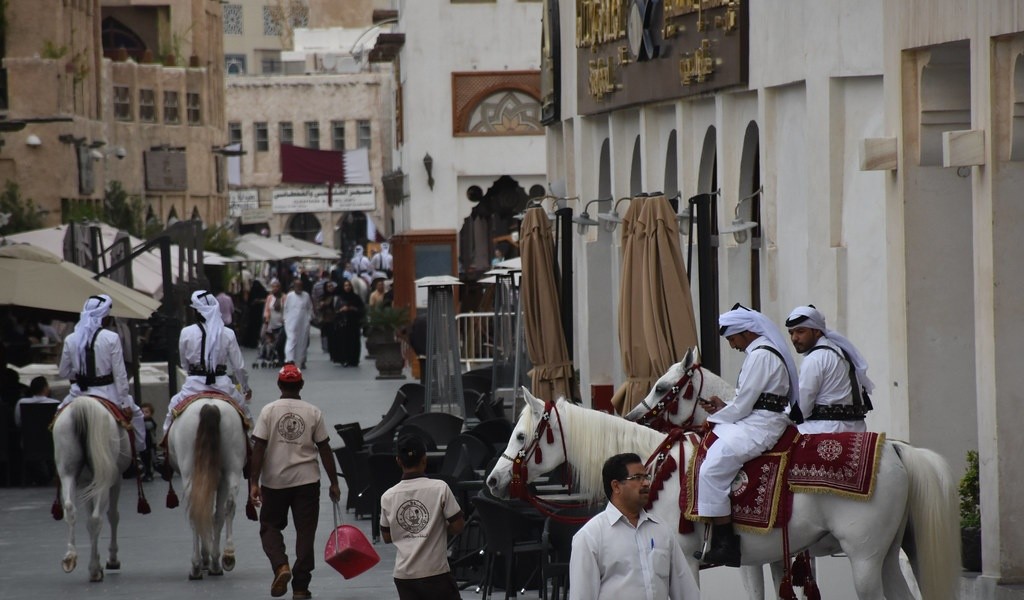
[620,474,651,483]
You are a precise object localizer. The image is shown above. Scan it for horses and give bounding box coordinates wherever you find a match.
[166,397,247,580]
[52,397,133,583]
[486,386,953,600]
[622,344,961,600]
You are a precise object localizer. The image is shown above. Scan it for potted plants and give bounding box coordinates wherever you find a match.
[958,449,983,572]
[364,301,409,379]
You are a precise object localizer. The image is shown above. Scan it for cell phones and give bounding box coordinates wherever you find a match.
[697,397,717,409]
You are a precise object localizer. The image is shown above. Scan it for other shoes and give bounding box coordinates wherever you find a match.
[152,459,173,482]
[242,463,250,479]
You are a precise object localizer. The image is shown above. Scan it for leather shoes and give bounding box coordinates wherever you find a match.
[292,589,311,600]
[271,564,291,597]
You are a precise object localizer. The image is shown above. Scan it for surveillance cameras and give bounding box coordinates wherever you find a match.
[115,146,126,159]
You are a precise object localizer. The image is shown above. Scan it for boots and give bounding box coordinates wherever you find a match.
[693,535,741,567]
[140,449,153,479]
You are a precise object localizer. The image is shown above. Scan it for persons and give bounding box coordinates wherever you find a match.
[569,452,701,600]
[238,243,391,368]
[379,434,465,600]
[139,405,158,482]
[697,305,799,567]
[16,376,61,438]
[156,289,255,450]
[250,364,340,600]
[492,250,505,266]
[214,287,234,326]
[55,294,145,450]
[786,304,870,434]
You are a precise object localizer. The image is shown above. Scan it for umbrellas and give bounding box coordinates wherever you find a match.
[521,205,582,402]
[1,215,226,320]
[238,233,341,260]
[612,191,700,420]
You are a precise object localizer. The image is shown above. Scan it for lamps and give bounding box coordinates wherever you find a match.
[511,191,566,223]
[112,145,127,159]
[596,196,632,235]
[422,153,436,189]
[349,17,399,64]
[88,149,103,162]
[571,196,614,237]
[24,133,42,148]
[721,186,764,243]
[673,182,722,238]
[545,191,578,228]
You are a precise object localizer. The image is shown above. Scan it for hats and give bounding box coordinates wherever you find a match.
[397,433,428,457]
[278,364,302,383]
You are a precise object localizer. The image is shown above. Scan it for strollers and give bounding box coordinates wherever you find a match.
[252,325,286,369]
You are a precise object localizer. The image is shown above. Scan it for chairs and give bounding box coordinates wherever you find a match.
[332,382,602,600]
[10,400,62,489]
